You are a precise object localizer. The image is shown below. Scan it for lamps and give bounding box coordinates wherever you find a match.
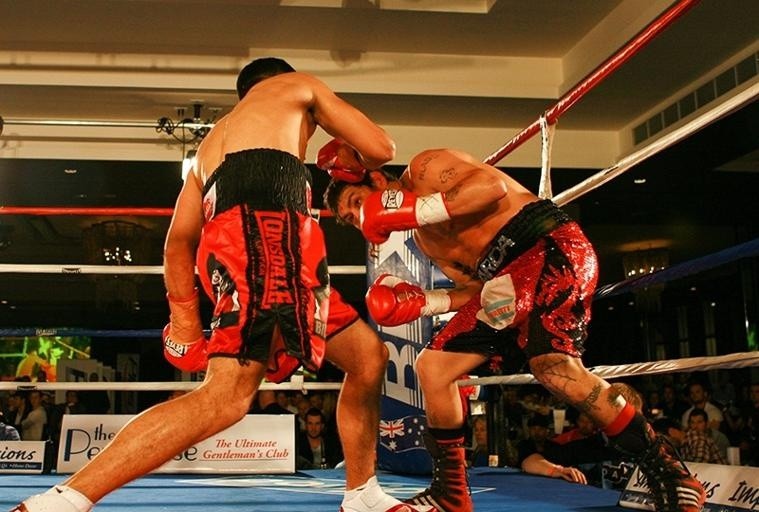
[176,99,217,182]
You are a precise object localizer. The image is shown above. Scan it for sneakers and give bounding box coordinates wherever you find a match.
[7,493,74,512]
[339,476,436,512]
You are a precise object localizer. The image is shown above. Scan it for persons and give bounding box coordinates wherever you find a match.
[6,55,441,512]
[0,365,759,492]
[323,146,712,511]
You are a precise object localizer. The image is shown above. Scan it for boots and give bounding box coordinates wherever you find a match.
[609,412,705,512]
[397,433,474,512]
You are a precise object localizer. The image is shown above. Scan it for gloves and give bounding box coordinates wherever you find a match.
[163,324,208,373]
[316,137,364,183]
[359,189,420,246]
[366,274,427,327]
